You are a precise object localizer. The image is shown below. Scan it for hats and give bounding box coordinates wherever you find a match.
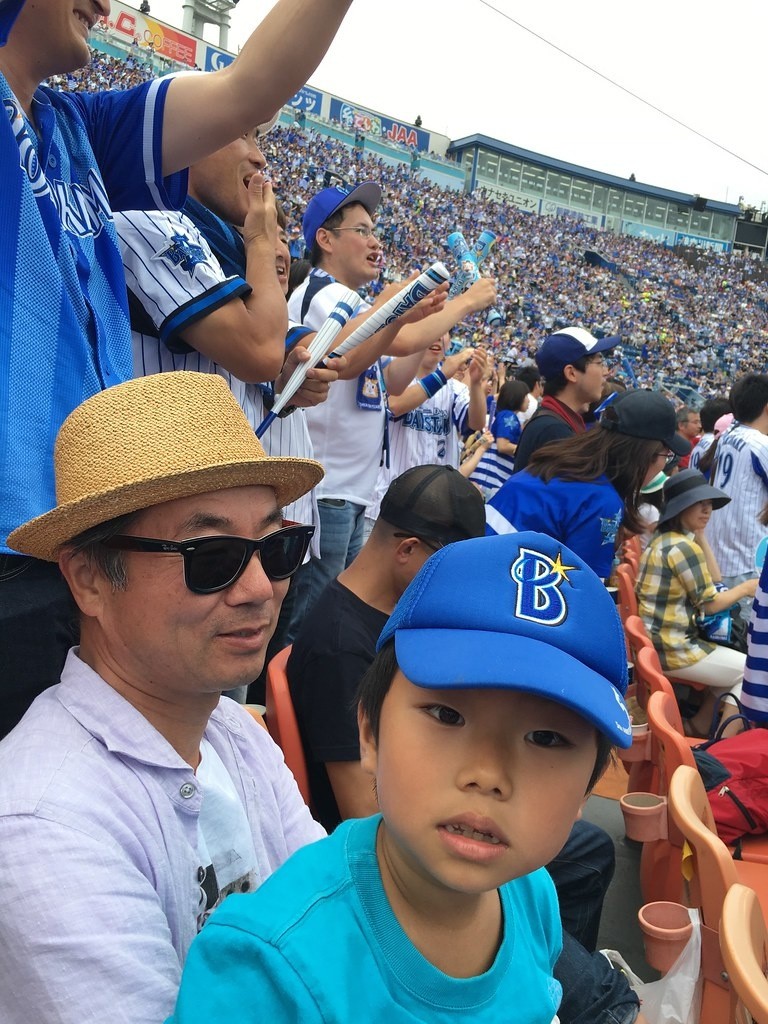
[6,370,324,564]
[714,413,734,440]
[380,463,487,545]
[602,387,691,456]
[376,531,633,750]
[654,466,731,531]
[535,326,622,381]
[304,181,381,252]
[639,470,670,494]
[162,70,280,138]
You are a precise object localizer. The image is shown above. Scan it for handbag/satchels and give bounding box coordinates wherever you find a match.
[689,692,768,847]
[599,907,702,1024]
[724,604,748,653]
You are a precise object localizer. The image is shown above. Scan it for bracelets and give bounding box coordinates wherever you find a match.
[418,368,447,399]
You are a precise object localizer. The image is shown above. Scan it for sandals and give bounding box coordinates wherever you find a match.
[682,719,709,739]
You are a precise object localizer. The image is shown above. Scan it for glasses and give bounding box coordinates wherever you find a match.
[329,226,374,238]
[585,358,607,369]
[86,519,315,594]
[654,450,676,465]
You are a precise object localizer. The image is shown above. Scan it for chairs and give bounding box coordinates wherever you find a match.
[267,529,768,1023]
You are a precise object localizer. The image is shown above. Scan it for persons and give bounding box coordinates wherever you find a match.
[166,531,633,1024]
[0,0,352,740]
[0,371,330,1024]
[288,464,649,1023]
[36,38,768,744]
